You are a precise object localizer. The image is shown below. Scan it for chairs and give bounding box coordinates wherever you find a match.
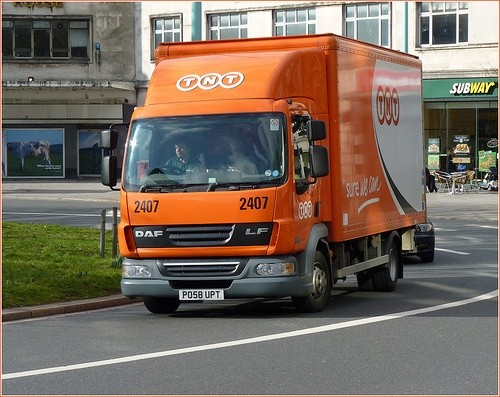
[435,170,475,194]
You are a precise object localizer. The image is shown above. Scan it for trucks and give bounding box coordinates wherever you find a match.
[99,31,426,314]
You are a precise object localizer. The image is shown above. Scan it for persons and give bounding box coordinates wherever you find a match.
[423,167,437,192]
[171,141,202,173]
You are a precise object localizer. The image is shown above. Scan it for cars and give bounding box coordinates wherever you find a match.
[401,215,436,263]
[478,172,497,192]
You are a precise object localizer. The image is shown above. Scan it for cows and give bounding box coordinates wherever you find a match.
[8,139,51,171]
